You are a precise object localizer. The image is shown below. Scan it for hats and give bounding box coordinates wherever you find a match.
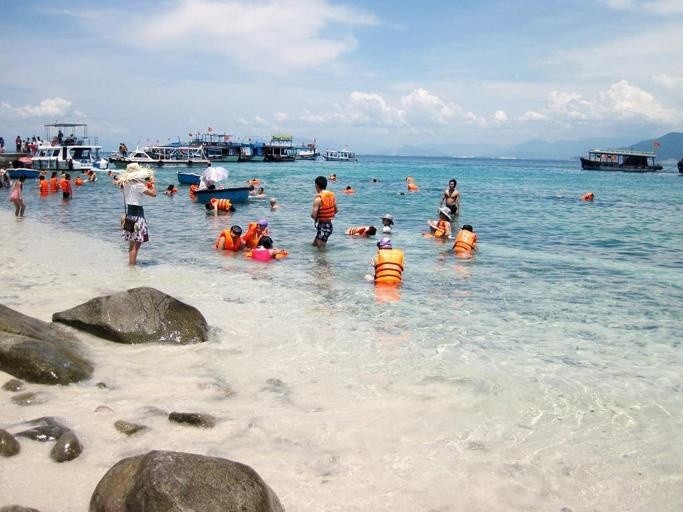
[379,212,396,226]
[116,161,153,185]
[438,206,454,222]
[256,219,269,227]
[380,237,391,249]
[269,197,276,203]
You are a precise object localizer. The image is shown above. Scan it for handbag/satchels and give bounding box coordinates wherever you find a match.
[124,218,136,233]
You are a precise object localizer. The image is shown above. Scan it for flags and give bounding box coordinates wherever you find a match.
[654,142,660,147]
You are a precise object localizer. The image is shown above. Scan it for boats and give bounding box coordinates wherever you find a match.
[194,185,258,206]
[676,158,683,177]
[579,149,664,173]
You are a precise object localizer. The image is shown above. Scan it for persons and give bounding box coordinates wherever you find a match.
[1,129,477,287]
[578,191,594,201]
